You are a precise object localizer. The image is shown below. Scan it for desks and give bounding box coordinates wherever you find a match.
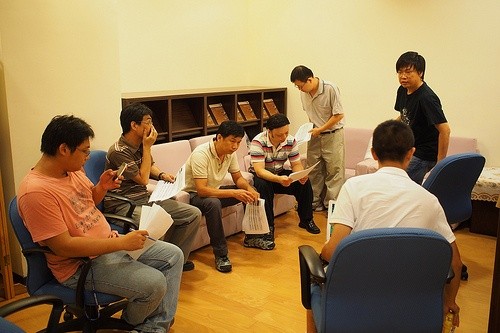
[470,167,500,237]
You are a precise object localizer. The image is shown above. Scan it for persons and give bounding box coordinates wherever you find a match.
[392,51,451,185]
[103,105,202,270]
[248,113,323,242]
[305,119,464,333]
[174,121,276,271]
[290,65,345,212]
[19,115,184,333]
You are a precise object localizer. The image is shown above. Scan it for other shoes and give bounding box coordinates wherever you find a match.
[295,203,323,211]
[182,262,194,272]
[215,254,232,273]
[244,236,275,250]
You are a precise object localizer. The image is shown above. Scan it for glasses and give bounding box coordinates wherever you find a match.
[294,82,306,89]
[137,120,153,126]
[397,73,412,77]
[77,148,91,157]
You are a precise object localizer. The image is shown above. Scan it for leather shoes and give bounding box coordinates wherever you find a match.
[298,218,320,234]
[264,226,274,242]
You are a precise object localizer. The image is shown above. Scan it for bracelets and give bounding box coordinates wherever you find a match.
[158,172,166,180]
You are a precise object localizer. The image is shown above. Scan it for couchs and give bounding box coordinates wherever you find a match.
[150,132,296,251]
[300,127,480,186]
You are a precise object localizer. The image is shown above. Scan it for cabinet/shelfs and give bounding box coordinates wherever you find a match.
[122,87,287,144]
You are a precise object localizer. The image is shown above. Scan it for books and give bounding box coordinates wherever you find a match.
[262,97,281,120]
[208,102,229,126]
[235,101,257,124]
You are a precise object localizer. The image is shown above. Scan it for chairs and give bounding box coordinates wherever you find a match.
[8,195,129,333]
[298,228,455,333]
[421,154,486,280]
[0,296,64,333]
[83,150,136,236]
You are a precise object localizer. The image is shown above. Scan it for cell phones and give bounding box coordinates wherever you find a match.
[112,162,128,181]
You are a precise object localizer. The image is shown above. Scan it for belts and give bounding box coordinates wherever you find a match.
[319,130,335,135]
